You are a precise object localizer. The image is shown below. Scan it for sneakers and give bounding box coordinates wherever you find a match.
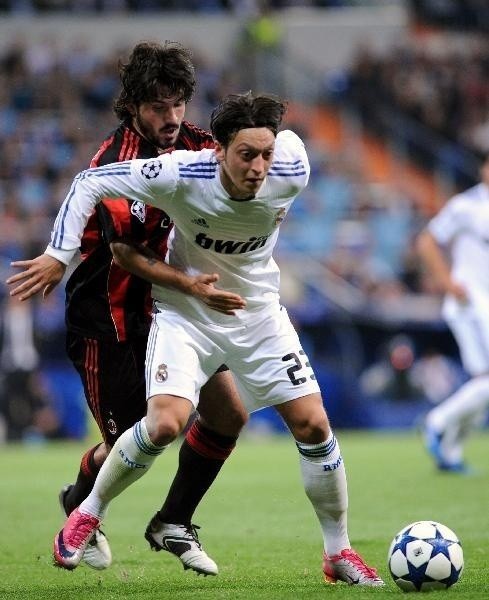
[145,513,219,577]
[61,486,111,570]
[322,549,386,586]
[54,503,101,568]
[426,410,467,472]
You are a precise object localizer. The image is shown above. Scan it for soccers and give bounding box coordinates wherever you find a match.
[388,520,465,592]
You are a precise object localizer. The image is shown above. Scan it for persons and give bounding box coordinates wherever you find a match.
[56,40,250,577]
[2,1,489,474]
[5,92,388,589]
